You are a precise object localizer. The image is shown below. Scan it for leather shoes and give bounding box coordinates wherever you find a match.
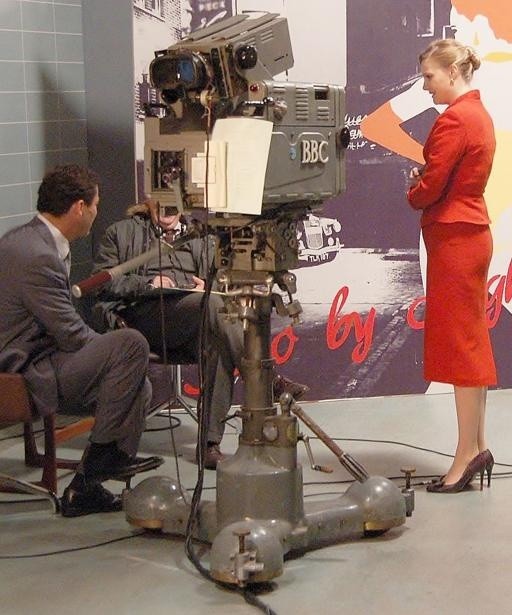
[114,456,165,477]
[196,445,225,470]
[62,484,122,517]
[273,375,309,400]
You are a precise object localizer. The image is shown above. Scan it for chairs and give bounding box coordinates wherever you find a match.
[116,313,201,425]
[0,370,96,515]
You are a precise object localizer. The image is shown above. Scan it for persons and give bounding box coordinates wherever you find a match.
[359,1,512,395]
[0,161,165,518]
[91,199,310,469]
[405,38,499,493]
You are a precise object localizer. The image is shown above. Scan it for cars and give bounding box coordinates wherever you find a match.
[296,213,344,263]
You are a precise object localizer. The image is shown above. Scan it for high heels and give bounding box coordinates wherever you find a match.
[426,449,495,493]
